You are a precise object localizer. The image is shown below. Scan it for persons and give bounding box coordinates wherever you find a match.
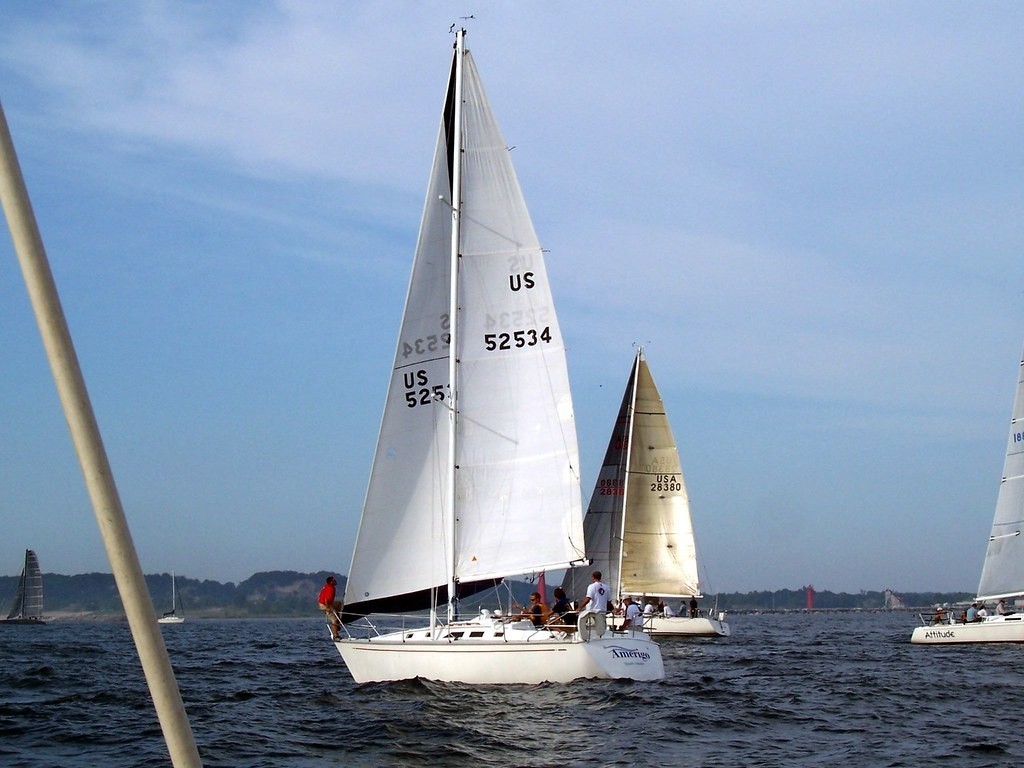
[319,578,343,642]
[935,600,1005,624]
[512,572,698,638]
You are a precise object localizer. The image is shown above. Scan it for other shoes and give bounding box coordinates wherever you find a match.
[333,636,342,642]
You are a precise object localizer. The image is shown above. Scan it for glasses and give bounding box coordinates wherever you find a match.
[530,598,537,602]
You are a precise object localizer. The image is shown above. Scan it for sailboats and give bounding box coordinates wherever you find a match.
[316,17,667,685]
[0,549,56,624]
[155,570,186,624]
[909,348,1024,644]
[549,340,731,637]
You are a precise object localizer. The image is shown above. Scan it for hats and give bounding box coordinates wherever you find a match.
[622,594,632,602]
[327,576,333,583]
